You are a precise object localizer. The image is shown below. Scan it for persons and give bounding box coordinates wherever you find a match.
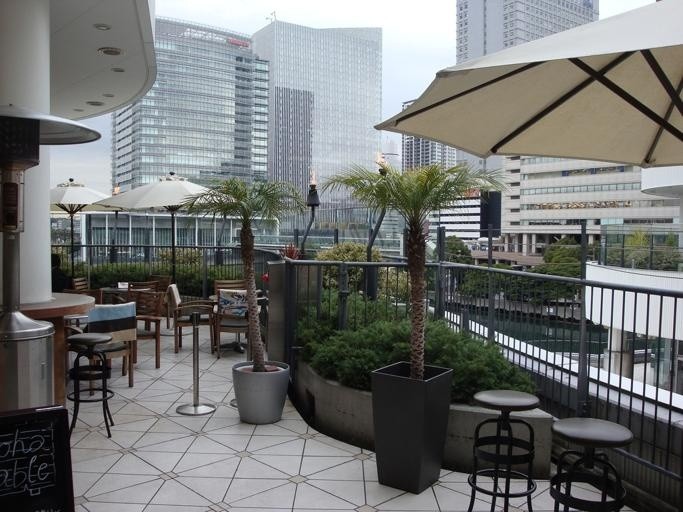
[51,253,73,292]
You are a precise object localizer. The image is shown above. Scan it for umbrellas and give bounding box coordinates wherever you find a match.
[93,171,237,283]
[375,2,683,170]
[50,178,110,278]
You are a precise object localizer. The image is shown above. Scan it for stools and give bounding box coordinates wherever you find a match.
[465,388,541,512]
[551,419,634,512]
[66,333,116,439]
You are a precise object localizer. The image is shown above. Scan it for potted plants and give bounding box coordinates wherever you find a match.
[179,176,311,425]
[316,155,512,495]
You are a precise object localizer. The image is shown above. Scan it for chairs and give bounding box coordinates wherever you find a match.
[62,289,80,327]
[129,290,166,369]
[85,302,136,394]
[212,279,248,346]
[166,284,216,355]
[76,289,103,330]
[213,288,252,362]
[73,277,88,296]
[145,275,173,331]
[112,281,158,316]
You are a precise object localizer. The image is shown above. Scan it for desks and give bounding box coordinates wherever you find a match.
[208,292,268,353]
[98,288,151,332]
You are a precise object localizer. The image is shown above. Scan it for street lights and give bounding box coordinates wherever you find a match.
[299,167,321,261]
[110,182,122,262]
[365,151,389,300]
